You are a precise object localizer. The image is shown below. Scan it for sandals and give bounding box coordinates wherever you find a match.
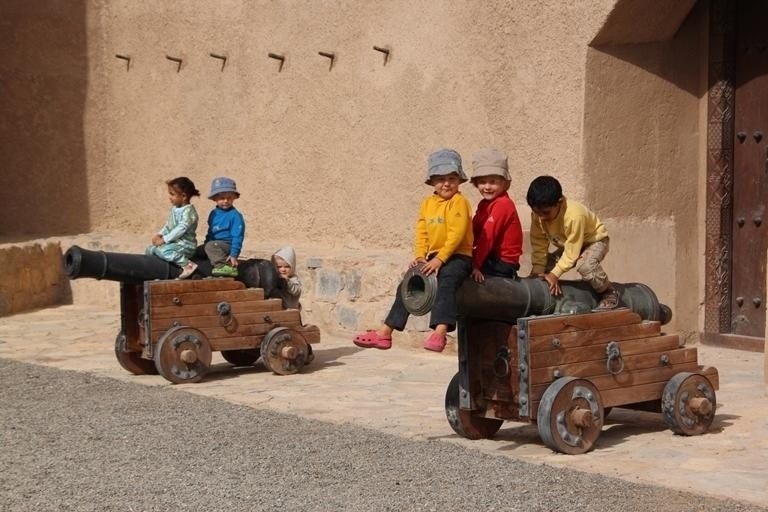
[598,282,621,308]
[423,332,447,352]
[353,329,391,349]
[212,264,238,277]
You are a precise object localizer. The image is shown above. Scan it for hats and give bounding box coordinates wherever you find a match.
[469,151,512,183]
[424,149,468,186]
[208,177,240,200]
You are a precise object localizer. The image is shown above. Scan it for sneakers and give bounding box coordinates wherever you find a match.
[304,354,314,364]
[179,260,198,278]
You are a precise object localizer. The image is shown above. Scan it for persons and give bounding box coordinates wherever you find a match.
[203,178,245,276]
[145,177,200,279]
[470,150,522,283]
[526,176,620,309]
[353,149,474,351]
[272,247,314,366]
